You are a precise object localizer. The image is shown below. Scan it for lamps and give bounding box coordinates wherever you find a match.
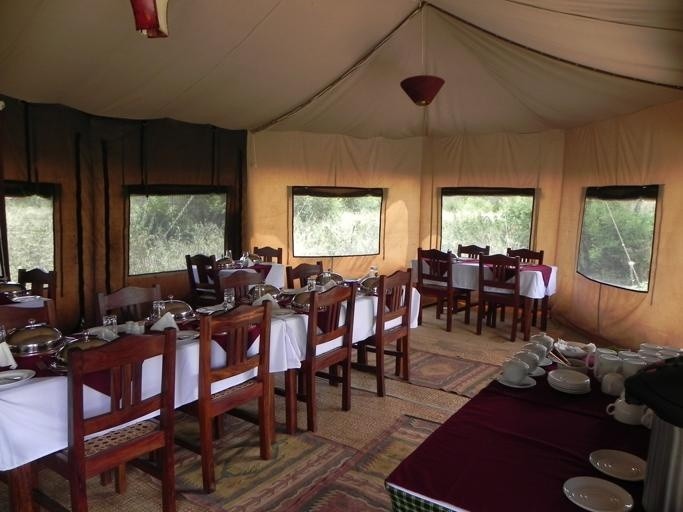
[399,1,445,107]
[130,0,169,39]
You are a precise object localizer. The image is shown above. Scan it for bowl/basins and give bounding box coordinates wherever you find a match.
[0,368,36,390]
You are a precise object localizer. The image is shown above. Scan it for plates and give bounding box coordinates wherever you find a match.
[554,341,590,359]
[547,368,592,395]
[272,309,297,319]
[494,374,537,390]
[497,367,547,378]
[588,448,646,481]
[536,357,553,367]
[13,295,39,302]
[562,475,635,511]
[175,329,200,344]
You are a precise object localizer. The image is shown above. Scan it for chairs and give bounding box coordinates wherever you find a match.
[439,244,490,318]
[335,269,413,396]
[286,261,325,289]
[95,283,162,325]
[477,253,525,342]
[215,269,265,303]
[34,327,176,512]
[276,282,357,431]
[500,248,544,327]
[253,244,281,263]
[174,301,277,493]
[186,254,221,306]
[416,247,472,332]
[17,268,57,311]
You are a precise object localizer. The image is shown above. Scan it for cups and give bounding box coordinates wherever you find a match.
[271,256,278,264]
[242,249,249,261]
[519,339,548,361]
[368,266,378,277]
[226,250,233,258]
[223,288,235,312]
[320,271,332,285]
[593,353,623,383]
[585,347,618,371]
[600,372,625,395]
[102,313,118,341]
[514,349,540,374]
[528,332,554,358]
[502,356,530,385]
[641,408,657,430]
[557,358,589,374]
[253,281,265,302]
[618,342,682,380]
[307,277,317,292]
[151,299,166,319]
[605,396,647,428]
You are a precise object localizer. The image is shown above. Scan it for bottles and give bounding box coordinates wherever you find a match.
[125,321,145,335]
